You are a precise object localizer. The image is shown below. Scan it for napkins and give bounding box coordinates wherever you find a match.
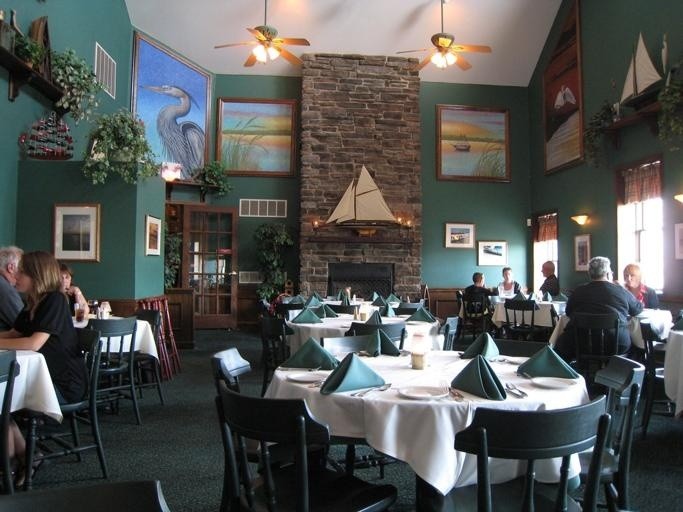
[319,352,385,395]
[516,343,579,379]
[450,353,507,401]
[459,332,500,359]
[279,336,340,370]
[366,328,401,358]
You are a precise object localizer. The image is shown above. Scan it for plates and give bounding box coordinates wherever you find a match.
[287,372,330,382]
[398,384,449,400]
[531,377,577,389]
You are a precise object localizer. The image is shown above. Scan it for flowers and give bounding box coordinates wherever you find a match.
[17,109,75,155]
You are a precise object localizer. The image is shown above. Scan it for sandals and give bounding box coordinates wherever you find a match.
[14,452,44,490]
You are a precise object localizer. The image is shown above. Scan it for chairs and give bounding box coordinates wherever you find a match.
[209,346,261,465]
[631,290,682,435]
[259,290,461,357]
[460,289,631,365]
[572,353,646,512]
[445,395,613,511]
[214,380,399,512]
[1,290,184,495]
[1,478,171,512]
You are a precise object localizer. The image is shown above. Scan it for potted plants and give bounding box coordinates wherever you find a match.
[584,98,620,168]
[11,34,56,68]
[79,108,162,186]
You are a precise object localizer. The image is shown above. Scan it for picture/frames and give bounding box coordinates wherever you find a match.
[477,240,508,267]
[145,213,161,256]
[215,97,298,179]
[435,103,512,183]
[51,203,101,263]
[673,222,683,261]
[444,221,476,249]
[573,234,592,273]
[129,30,212,182]
[542,0,585,176]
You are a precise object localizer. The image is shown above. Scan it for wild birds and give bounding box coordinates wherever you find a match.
[138,84,205,178]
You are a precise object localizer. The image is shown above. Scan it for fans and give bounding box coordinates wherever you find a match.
[395,0,492,72]
[213,0,312,67]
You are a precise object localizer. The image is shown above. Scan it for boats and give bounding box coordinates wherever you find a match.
[454,143,471,150]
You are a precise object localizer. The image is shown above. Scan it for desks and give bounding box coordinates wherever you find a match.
[264,351,591,512]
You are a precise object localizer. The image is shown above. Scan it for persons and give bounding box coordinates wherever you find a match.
[0,246,24,332]
[622,263,659,310]
[0,250,86,494]
[539,261,559,297]
[565,256,645,360]
[465,272,499,334]
[496,267,520,298]
[60,263,90,319]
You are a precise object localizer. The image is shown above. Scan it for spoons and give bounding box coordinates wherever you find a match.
[506,382,528,397]
[357,384,391,398]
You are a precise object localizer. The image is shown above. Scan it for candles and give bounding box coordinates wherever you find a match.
[313,221,318,227]
[406,220,411,227]
[398,217,402,224]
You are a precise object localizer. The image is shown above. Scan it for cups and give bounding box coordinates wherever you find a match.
[75,303,85,322]
[88,299,98,314]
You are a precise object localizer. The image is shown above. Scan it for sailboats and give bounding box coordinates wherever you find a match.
[326,164,400,230]
[619,29,671,107]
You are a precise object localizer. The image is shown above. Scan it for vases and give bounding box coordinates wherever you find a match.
[29,152,72,160]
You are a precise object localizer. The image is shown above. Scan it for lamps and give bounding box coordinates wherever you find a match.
[251,39,280,64]
[431,48,459,69]
[569,213,589,226]
[673,193,683,203]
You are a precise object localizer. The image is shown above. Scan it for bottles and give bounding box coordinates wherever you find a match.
[411,334,425,369]
